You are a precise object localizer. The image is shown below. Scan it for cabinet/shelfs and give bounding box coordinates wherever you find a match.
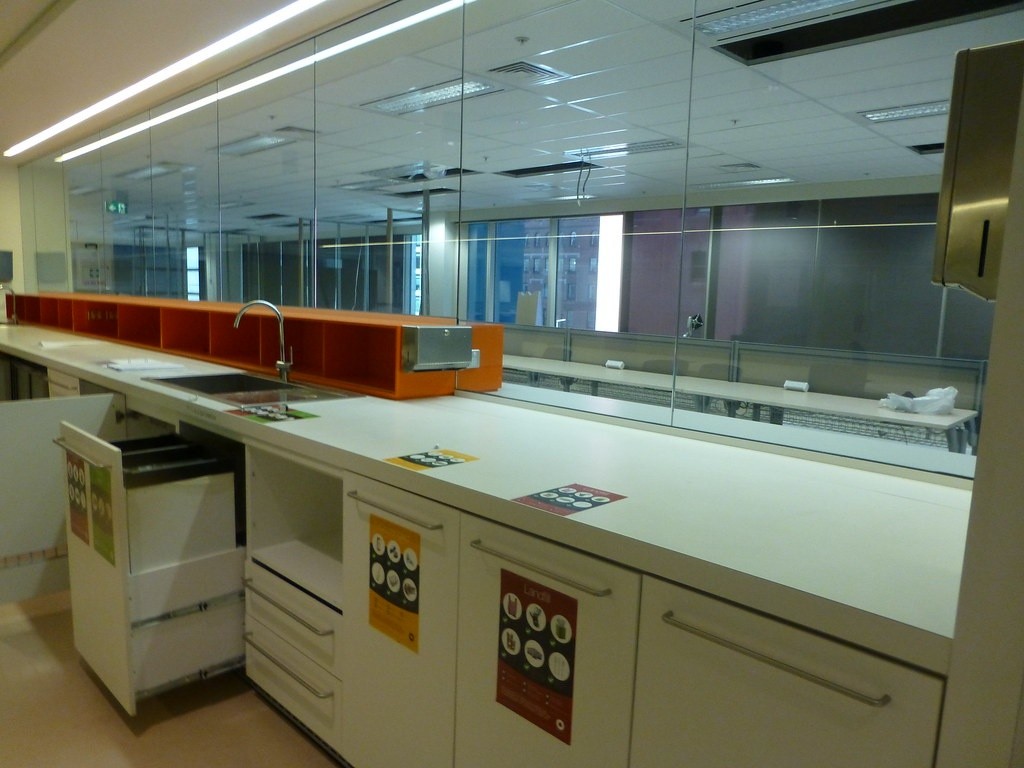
[0,325,948,766]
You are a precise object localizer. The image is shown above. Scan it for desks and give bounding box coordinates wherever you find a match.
[501,351,979,451]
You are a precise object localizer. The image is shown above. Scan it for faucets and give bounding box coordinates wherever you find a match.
[0,284,20,327]
[232,298,295,384]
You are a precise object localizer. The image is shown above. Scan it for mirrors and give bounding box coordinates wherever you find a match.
[17,0,1024,482]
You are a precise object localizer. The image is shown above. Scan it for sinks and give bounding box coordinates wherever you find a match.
[207,388,367,409]
[139,371,312,397]
